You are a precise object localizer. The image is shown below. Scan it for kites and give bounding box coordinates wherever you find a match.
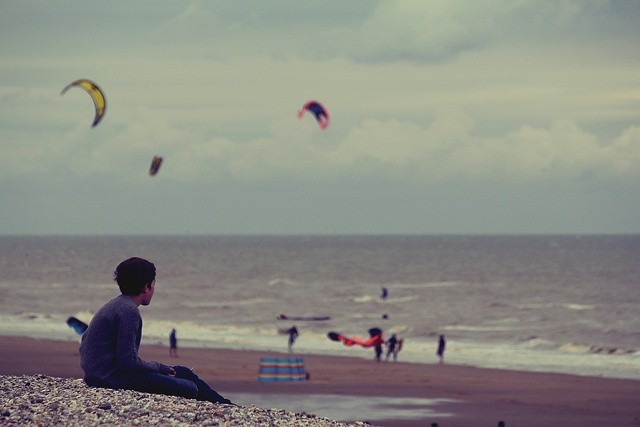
[327,327,385,349]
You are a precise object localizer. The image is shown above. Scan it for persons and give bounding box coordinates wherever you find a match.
[369,327,382,359]
[287,326,298,353]
[385,334,397,361]
[80,258,236,405]
[437,335,446,361]
[170,328,178,358]
